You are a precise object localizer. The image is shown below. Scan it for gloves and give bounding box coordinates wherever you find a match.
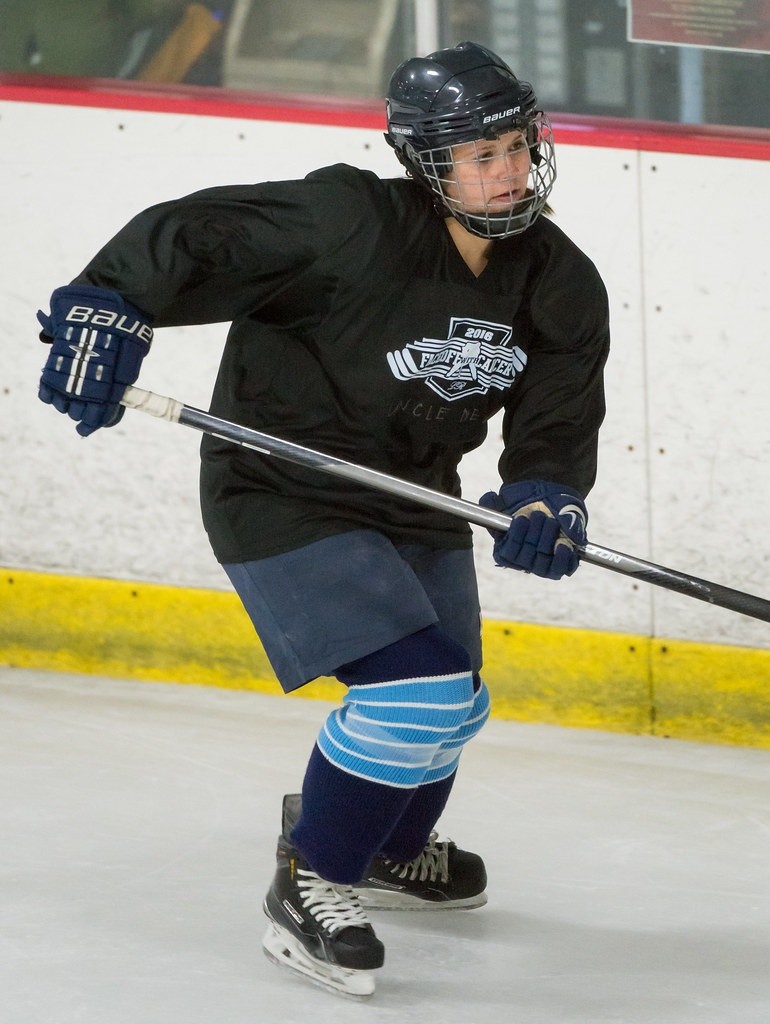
[478,479,589,581]
[37,285,153,437]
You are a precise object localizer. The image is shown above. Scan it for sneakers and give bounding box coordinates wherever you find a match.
[262,794,385,1001]
[354,829,489,913]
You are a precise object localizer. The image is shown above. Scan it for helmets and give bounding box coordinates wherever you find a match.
[383,40,558,240]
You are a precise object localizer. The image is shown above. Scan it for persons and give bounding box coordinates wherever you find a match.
[37,40,610,1005]
